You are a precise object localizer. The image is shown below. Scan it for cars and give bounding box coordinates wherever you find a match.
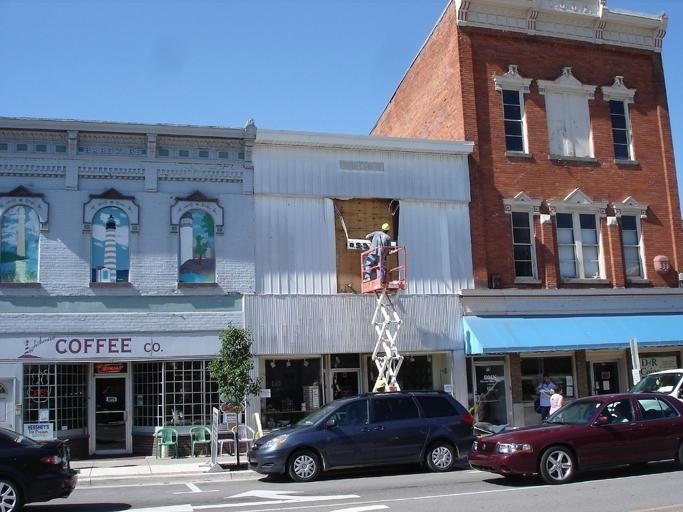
[0,427,78,512]
[468,392,683,485]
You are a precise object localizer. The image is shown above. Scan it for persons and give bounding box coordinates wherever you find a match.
[605,405,631,424]
[535,372,556,421]
[361,222,392,284]
[548,386,564,421]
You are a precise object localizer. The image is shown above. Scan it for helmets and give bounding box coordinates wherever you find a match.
[382,222,390,231]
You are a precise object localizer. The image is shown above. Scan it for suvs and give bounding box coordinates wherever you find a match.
[248,383,478,481]
[597,370,683,421]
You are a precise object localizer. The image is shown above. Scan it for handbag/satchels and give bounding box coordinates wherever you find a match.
[533,398,542,414]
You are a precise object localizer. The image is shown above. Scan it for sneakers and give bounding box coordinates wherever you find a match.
[363,277,371,283]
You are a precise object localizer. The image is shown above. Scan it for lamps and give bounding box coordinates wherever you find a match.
[269,358,309,369]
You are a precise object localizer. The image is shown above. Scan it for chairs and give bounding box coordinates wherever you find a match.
[154,423,256,460]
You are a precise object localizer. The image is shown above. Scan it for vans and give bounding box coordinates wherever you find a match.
[479,375,566,397]
